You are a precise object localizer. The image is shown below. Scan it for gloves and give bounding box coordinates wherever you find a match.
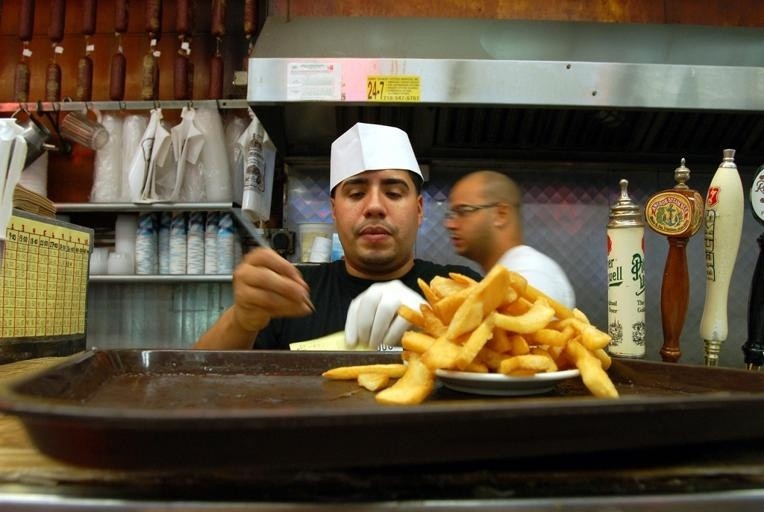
[344,281,433,352]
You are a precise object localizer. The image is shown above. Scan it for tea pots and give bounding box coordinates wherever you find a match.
[10,109,58,171]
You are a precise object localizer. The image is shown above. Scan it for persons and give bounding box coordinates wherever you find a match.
[192,123,483,350]
[443,170,576,321]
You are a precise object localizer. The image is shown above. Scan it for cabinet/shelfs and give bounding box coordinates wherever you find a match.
[1,100,250,283]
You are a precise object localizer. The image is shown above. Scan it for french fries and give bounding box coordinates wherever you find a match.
[322,265,620,407]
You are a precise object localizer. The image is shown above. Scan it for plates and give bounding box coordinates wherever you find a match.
[433,369,581,397]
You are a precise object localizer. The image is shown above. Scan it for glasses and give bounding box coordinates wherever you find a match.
[443,202,498,219]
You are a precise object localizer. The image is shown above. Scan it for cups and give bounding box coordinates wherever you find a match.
[135,212,244,276]
[56,105,111,152]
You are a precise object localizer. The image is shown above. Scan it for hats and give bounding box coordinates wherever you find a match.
[330,122,424,193]
